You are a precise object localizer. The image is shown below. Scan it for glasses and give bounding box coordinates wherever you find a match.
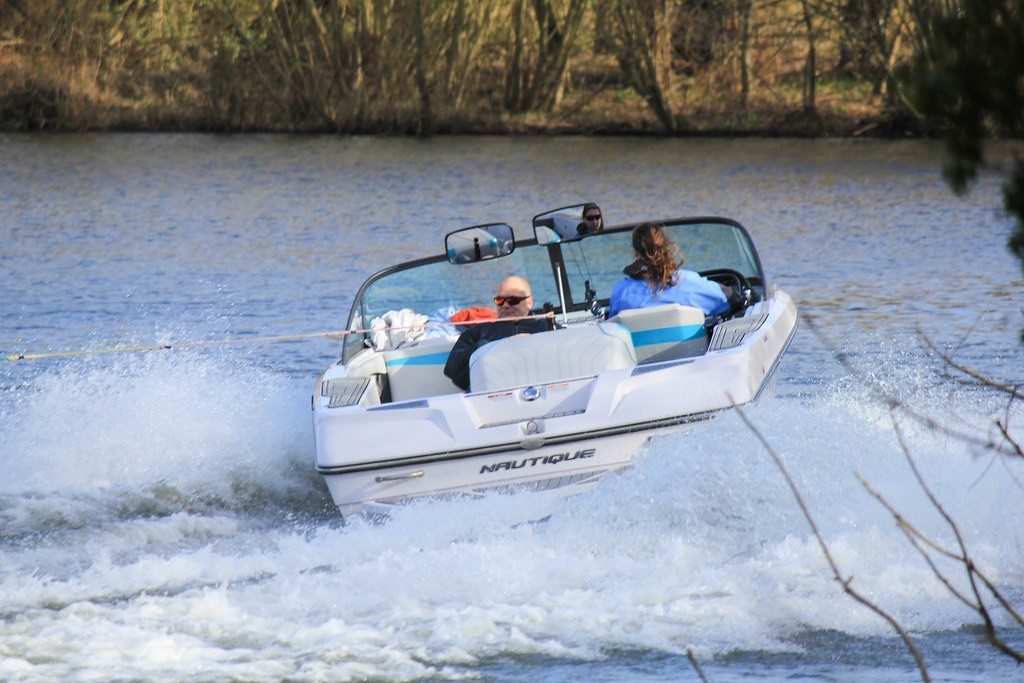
[494,296,532,306]
[584,214,600,221]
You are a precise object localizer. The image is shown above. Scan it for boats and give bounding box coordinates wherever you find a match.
[309,201,800,526]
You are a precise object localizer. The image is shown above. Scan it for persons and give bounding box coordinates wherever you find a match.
[574,204,603,238]
[608,223,728,318]
[444,276,550,392]
